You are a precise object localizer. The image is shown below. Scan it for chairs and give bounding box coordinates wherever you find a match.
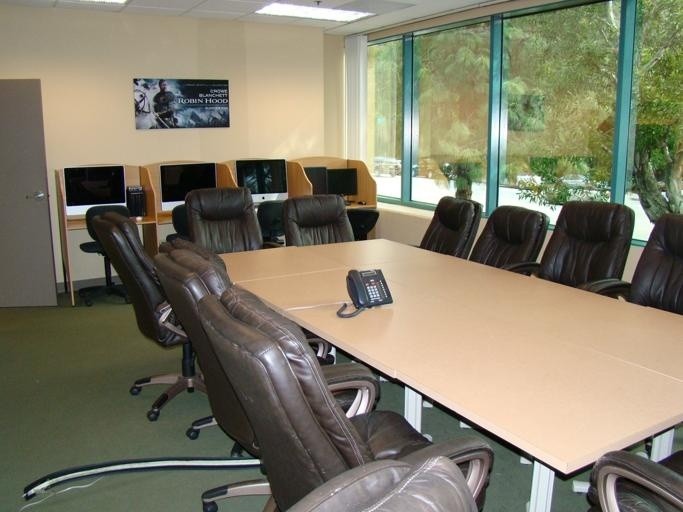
[585,213,682,315]
[152,236,338,458]
[407,194,484,258]
[582,442,683,510]
[279,452,480,512]
[194,283,495,511]
[85,210,203,422]
[498,200,636,289]
[465,205,550,271]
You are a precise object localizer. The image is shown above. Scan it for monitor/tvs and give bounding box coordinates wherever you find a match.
[328,168,357,196]
[235,158,288,202]
[159,162,217,212]
[62,164,126,215]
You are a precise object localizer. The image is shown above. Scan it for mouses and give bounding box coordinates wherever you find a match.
[136,216,143,221]
[358,201,362,205]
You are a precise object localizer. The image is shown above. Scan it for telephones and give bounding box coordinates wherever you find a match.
[346,269,393,309]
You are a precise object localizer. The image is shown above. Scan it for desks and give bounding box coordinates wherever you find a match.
[214,237,682,510]
